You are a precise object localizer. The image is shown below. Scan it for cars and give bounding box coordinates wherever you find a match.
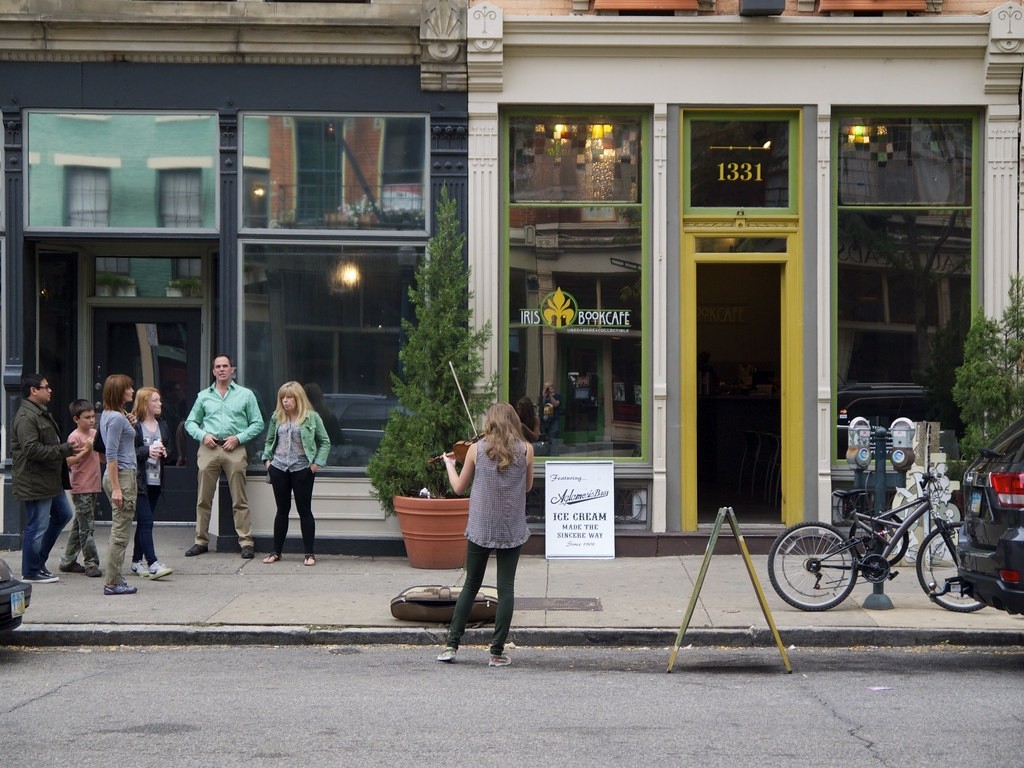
[0,560,33,637]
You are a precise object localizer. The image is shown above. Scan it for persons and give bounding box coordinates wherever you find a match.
[261,380,331,566]
[102,374,138,595]
[516,397,540,443]
[59,399,102,577]
[131,387,174,579]
[303,383,345,465]
[184,354,265,558]
[536,382,562,444]
[438,402,534,667]
[10,374,85,583]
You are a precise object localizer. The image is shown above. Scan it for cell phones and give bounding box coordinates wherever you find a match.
[153,441,162,458]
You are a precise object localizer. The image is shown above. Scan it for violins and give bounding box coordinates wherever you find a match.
[426,439,474,465]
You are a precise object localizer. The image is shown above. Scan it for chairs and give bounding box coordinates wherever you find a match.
[736,428,780,511]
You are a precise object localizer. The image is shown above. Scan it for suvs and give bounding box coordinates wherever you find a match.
[322,393,401,452]
[957,409,1023,616]
[836,383,964,457]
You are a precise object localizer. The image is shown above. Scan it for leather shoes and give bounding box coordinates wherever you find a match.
[241,546,255,558]
[185,544,209,556]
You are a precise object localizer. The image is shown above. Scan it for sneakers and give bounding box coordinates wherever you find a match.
[104,583,137,595]
[437,647,456,661]
[85,565,102,576]
[59,561,85,572]
[148,562,174,580]
[488,653,510,665]
[20,572,59,583]
[130,562,149,576]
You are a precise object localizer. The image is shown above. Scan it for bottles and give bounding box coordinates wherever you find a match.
[147,439,162,464]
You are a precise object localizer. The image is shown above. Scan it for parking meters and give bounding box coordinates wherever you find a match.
[847,417,915,611]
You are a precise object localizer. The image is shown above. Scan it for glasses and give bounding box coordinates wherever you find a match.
[36,385,50,390]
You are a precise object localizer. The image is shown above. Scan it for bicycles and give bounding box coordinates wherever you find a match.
[767,473,986,612]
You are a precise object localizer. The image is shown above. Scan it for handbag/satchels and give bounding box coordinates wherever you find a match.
[94,410,127,453]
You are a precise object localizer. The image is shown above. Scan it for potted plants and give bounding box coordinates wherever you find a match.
[95,271,136,297]
[165,277,202,298]
[367,182,505,568]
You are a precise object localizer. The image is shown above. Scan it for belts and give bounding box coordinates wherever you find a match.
[214,440,226,445]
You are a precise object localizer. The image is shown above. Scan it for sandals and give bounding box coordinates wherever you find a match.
[305,554,316,565]
[264,552,283,563]
